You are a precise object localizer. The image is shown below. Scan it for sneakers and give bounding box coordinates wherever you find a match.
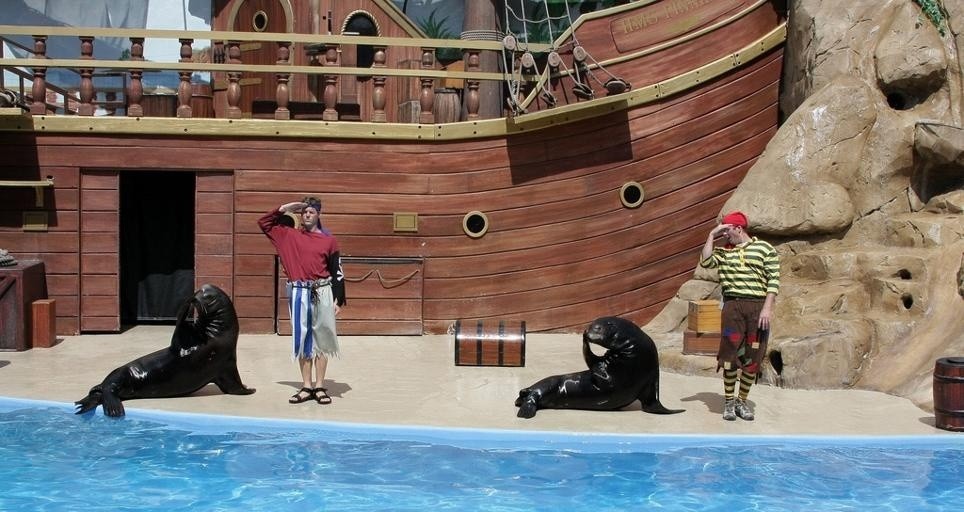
[724,400,737,420]
[735,398,754,420]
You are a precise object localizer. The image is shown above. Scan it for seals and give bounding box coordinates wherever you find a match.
[515,315,683,422]
[71,283,258,421]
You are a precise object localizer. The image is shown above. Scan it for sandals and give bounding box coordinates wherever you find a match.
[289,387,314,403]
[313,388,331,404]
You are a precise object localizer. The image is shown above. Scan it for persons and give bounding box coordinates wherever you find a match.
[258,196,345,404]
[693,211,781,420]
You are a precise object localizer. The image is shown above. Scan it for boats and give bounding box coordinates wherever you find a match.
[0,3,797,342]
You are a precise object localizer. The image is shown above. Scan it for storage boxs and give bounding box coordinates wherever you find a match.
[682,330,726,356]
[687,298,724,334]
